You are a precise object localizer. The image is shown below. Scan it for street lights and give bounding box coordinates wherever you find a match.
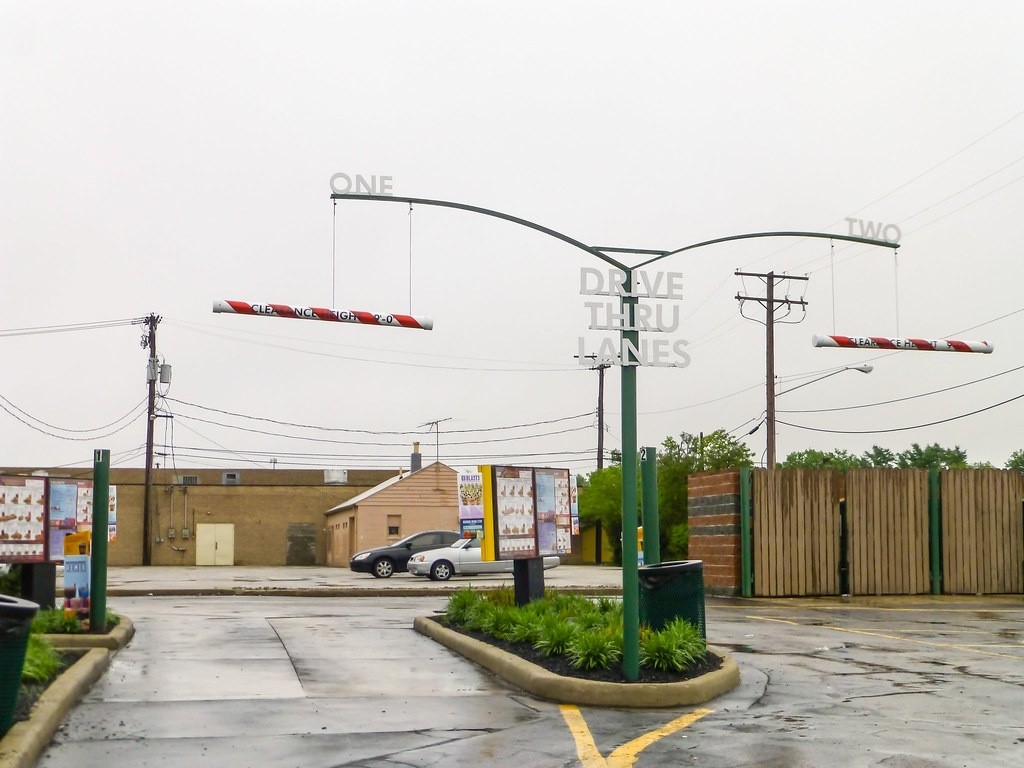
[766,365,875,469]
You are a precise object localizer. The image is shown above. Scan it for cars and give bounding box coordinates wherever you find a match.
[407,538,560,581]
[349,529,461,578]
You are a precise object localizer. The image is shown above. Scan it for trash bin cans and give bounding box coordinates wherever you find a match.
[637,558,707,653]
[0,596,42,737]
